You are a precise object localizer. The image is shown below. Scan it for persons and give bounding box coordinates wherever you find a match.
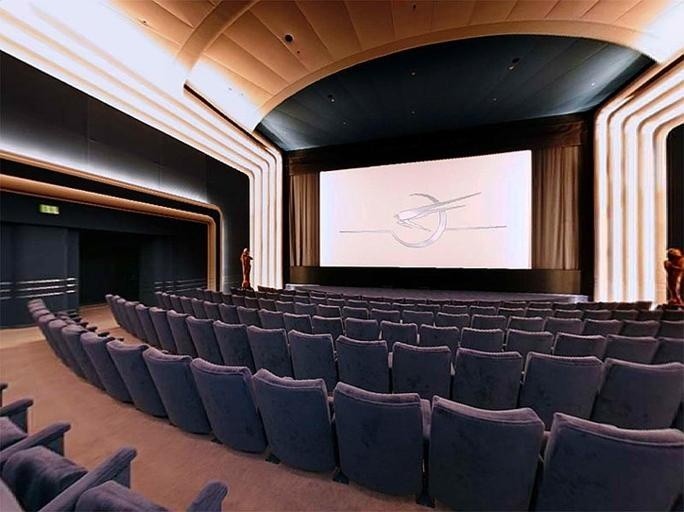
[239,247,253,289]
[663,247,684,305]
